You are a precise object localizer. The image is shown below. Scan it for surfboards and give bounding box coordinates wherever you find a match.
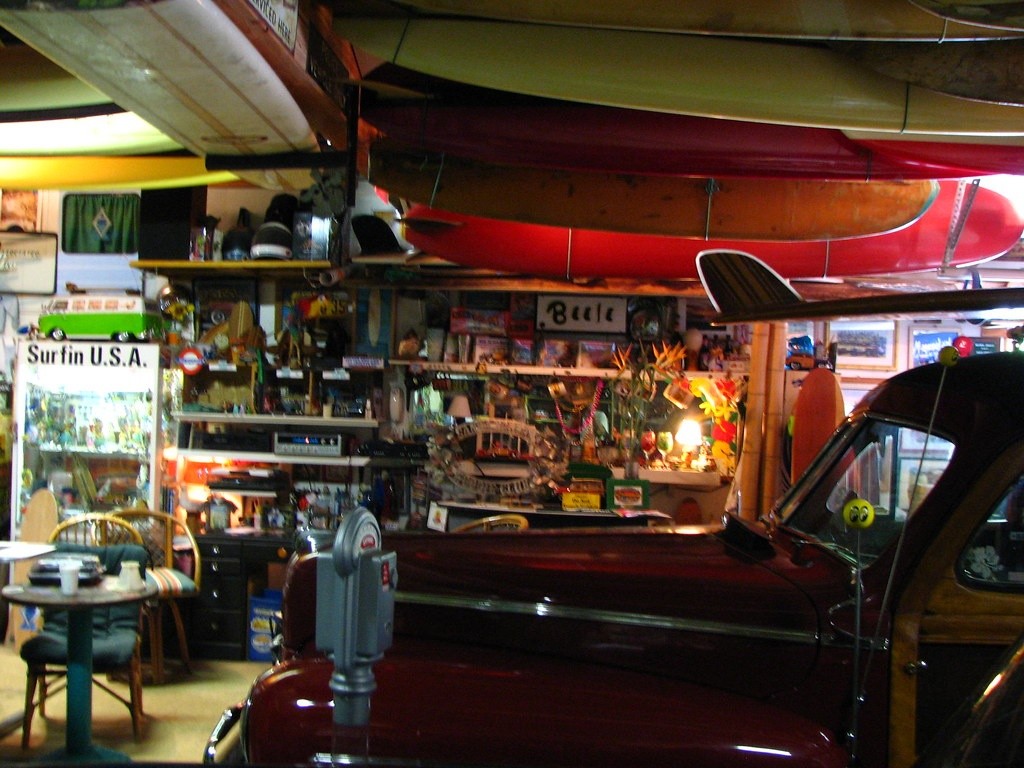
[811,39,1024,108]
[331,0,1024,136]
[338,61,1024,181]
[385,0,1024,45]
[367,137,940,244]
[0,0,324,193]
[393,179,1023,280]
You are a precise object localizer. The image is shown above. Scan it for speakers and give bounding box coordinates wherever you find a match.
[138,185,208,261]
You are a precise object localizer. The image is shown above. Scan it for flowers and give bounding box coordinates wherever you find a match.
[607,336,689,463]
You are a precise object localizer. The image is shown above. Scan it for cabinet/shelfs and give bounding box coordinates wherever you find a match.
[188,530,296,661]
[128,257,842,486]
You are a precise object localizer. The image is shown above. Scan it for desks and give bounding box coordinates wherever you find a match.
[2,574,160,767]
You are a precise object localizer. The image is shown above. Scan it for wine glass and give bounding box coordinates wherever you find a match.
[656,431,674,470]
[641,430,656,471]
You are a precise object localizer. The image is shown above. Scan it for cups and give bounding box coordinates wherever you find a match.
[117,561,146,592]
[59,558,80,595]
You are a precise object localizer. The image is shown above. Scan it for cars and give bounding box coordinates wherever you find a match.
[203,348,1024,768]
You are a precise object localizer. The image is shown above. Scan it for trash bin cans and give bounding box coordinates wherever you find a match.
[248,587,285,661]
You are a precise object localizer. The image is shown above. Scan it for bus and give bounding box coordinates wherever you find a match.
[36,288,165,345]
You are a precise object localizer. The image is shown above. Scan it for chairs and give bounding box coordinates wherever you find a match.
[90,508,201,685]
[20,514,146,747]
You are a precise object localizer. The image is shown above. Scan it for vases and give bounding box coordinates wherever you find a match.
[622,448,639,480]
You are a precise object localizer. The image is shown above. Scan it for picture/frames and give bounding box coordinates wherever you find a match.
[908,326,960,369]
[824,320,900,371]
[788,320,819,345]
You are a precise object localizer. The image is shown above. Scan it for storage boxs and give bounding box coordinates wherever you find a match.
[605,478,650,511]
[246,587,283,662]
[452,295,629,367]
[557,485,602,513]
